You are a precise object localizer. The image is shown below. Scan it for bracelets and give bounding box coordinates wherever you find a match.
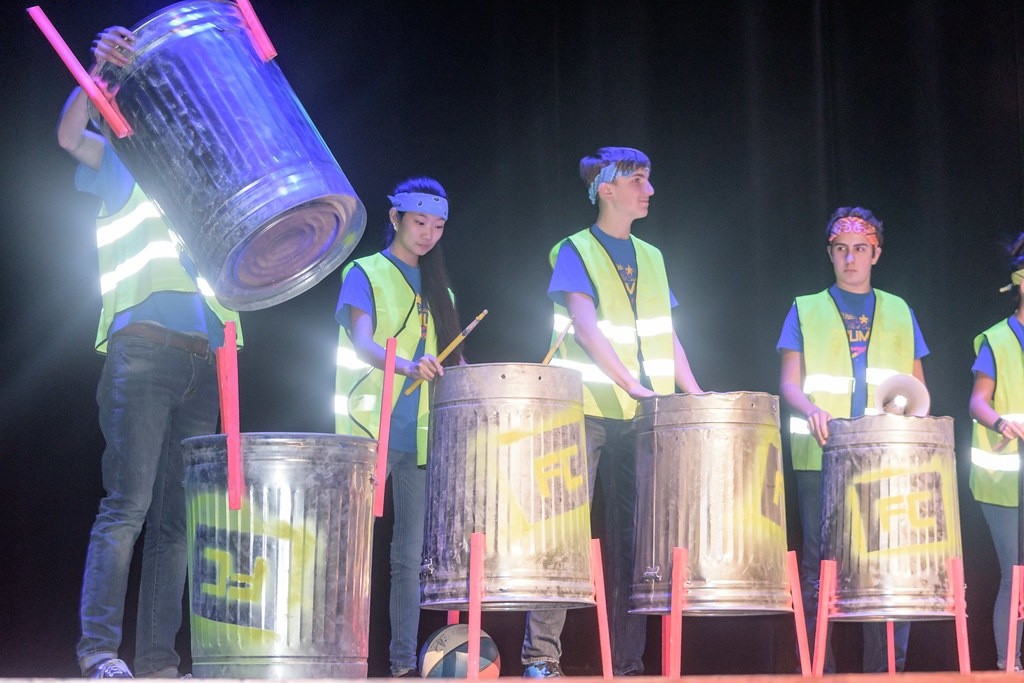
[993,418,1007,433]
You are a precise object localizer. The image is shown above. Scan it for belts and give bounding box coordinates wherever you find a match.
[111,322,218,361]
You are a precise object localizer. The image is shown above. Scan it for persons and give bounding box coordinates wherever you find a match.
[777,206,929,678]
[967,234,1024,673]
[57,25,243,679]
[335,178,468,679]
[518,147,707,679]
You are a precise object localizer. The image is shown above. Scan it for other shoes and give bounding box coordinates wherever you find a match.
[88,658,134,678]
[391,668,422,678]
[523,662,565,678]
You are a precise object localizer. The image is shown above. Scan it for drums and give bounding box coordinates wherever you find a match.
[84,0,370,313]
[626,390,798,617]
[813,412,971,624]
[418,360,600,613]
[177,431,380,679]
